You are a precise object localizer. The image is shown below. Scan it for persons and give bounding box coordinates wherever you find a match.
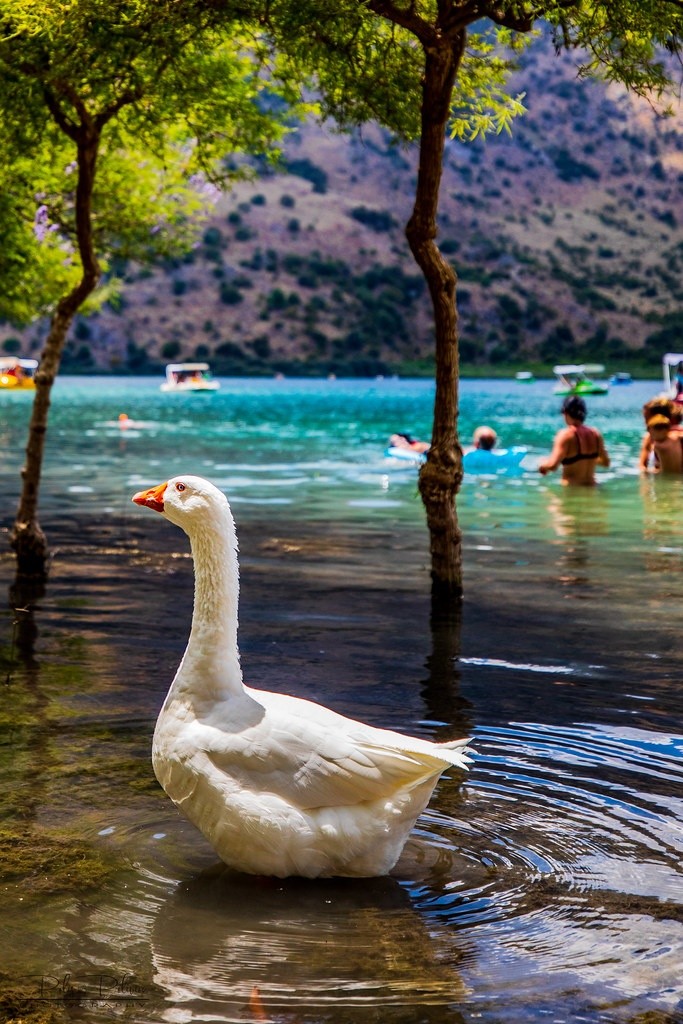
[538,395,611,486]
[390,425,497,457]
[638,361,683,474]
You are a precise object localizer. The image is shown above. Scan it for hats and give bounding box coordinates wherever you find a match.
[560,395,587,420]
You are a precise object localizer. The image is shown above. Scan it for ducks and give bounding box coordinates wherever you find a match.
[132,474,481,879]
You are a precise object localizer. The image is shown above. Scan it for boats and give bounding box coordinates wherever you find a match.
[513,370,535,383]
[657,350,683,409]
[0,357,38,388]
[160,362,221,394]
[554,362,612,397]
[608,372,635,387]
